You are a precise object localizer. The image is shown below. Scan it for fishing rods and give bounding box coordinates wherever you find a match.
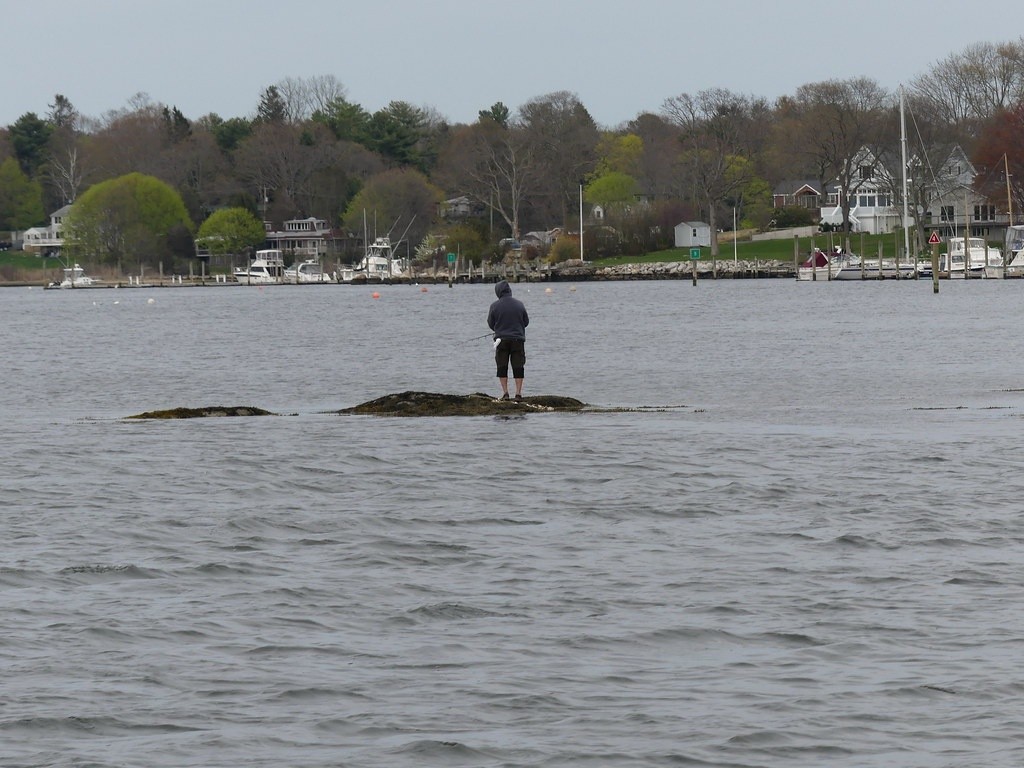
[454,333,493,347]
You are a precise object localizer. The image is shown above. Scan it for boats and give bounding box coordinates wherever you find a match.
[43,263,104,289]
[795,219,1024,281]
[232,249,332,286]
[339,236,415,284]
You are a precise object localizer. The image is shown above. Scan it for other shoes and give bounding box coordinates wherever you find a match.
[513,395,522,404]
[491,394,509,402]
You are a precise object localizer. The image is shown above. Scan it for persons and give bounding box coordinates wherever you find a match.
[488,281,528,400]
